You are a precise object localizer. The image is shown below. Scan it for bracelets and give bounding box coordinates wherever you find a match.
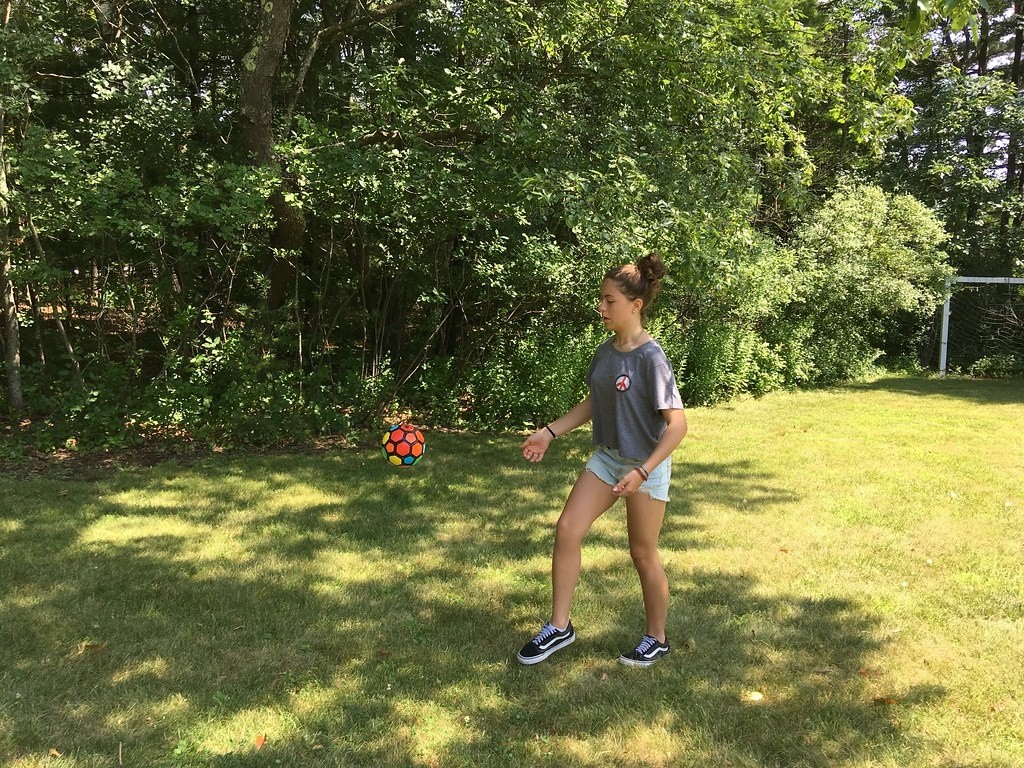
[634,467,647,481]
[545,425,556,439]
[639,465,649,477]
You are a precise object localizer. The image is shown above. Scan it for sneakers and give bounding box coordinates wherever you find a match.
[516,621,575,665]
[618,635,670,667]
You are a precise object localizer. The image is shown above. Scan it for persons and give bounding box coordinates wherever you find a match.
[516,254,688,668]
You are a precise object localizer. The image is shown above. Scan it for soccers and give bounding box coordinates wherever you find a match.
[381,424,426,470]
